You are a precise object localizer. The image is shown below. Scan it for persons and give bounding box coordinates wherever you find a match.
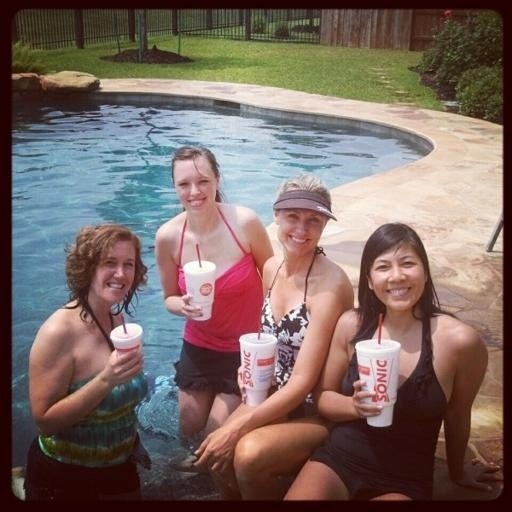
[154,145,275,454]
[192,170,357,502]
[283,220,503,503]
[18,222,152,503]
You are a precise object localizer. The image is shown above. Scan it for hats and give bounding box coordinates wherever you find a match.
[273,191,338,222]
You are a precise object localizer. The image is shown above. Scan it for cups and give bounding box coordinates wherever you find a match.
[239,331,278,406]
[354,339,402,428]
[184,260,216,320]
[109,323,143,356]
